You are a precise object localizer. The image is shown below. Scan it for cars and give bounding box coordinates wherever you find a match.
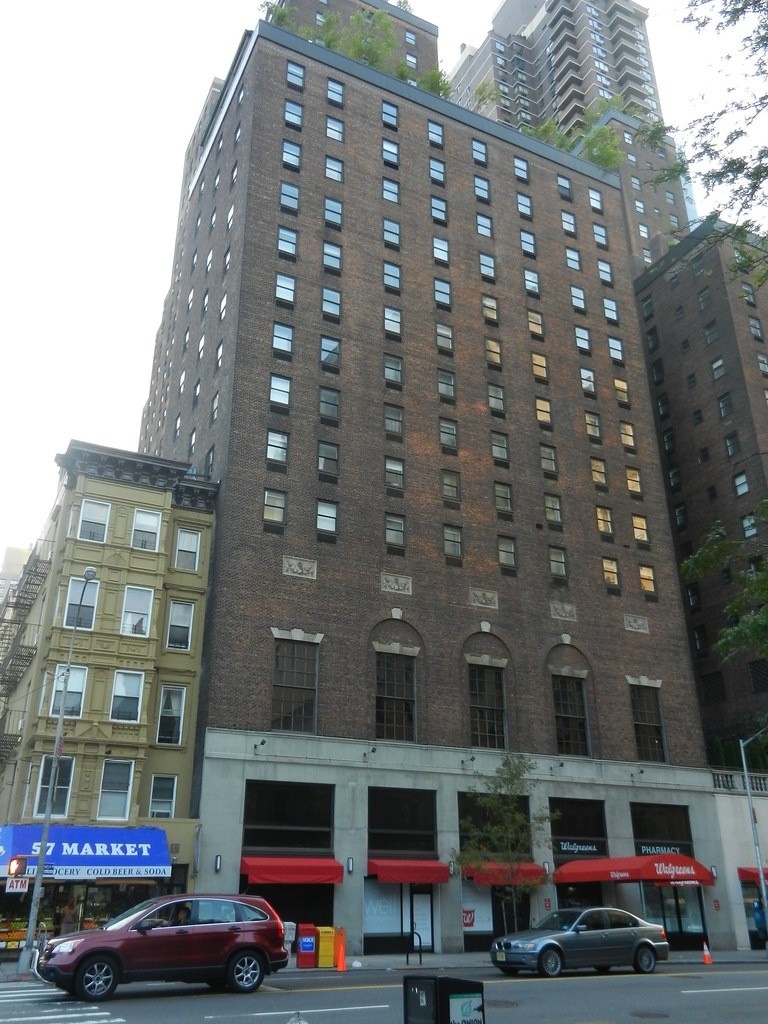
[489,906,669,977]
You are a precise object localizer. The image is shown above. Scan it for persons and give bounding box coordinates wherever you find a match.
[178,906,191,926]
[59,896,78,936]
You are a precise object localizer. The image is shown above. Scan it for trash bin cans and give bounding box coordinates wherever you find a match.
[403,975,485,1024]
[283,922,335,969]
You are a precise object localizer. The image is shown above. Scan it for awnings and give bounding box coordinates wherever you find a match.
[368,858,450,883]
[240,858,345,884]
[0,823,172,878]
[554,853,715,922]
[462,861,549,886]
[737,867,768,887]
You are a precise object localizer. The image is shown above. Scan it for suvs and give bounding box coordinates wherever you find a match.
[37,893,289,1002]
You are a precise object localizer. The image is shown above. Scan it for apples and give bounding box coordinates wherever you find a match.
[11,922,26,929]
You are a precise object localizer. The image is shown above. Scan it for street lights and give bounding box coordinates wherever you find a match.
[17,568,99,974]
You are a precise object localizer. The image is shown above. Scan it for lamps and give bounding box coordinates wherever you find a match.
[543,861,549,876]
[630,769,644,777]
[550,762,564,772]
[364,747,376,756]
[348,857,354,872]
[711,865,718,878]
[216,855,221,871]
[449,860,455,876]
[253,738,267,748]
[461,756,475,765]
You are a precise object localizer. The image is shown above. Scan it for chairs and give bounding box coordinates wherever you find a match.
[166,905,180,926]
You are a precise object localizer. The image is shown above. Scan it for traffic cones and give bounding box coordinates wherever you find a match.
[336,944,347,971]
[703,941,713,964]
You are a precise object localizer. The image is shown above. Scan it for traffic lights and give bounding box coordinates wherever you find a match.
[8,858,27,875]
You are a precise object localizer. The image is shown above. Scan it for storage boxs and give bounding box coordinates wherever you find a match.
[7,941,19,949]
[0,942,6,948]
